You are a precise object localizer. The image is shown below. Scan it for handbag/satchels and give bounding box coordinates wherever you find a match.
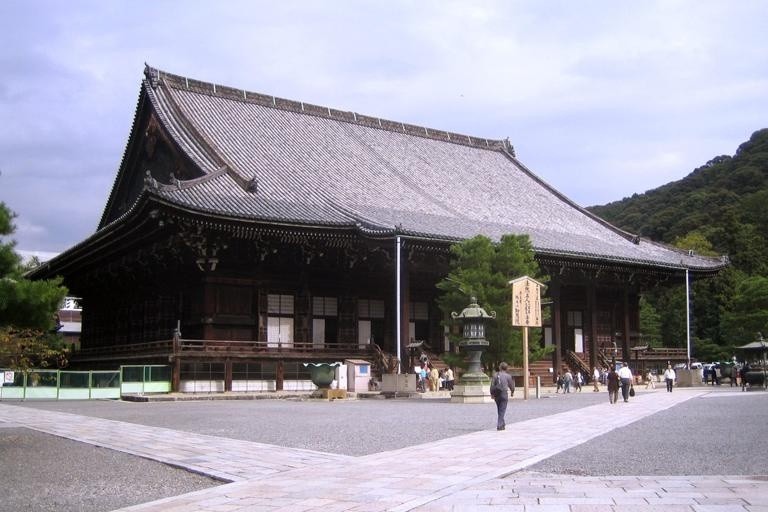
[617,381,623,387]
[490,374,503,395]
[630,387,634,396]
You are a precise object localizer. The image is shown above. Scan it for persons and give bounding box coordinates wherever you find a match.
[489,361,515,431]
[553,361,633,404]
[710,366,717,386]
[643,370,655,390]
[715,364,721,385]
[740,363,750,393]
[407,349,454,393]
[701,365,709,385]
[662,364,676,393]
[729,365,737,388]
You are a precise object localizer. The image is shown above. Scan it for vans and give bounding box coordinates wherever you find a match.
[674,362,716,381]
